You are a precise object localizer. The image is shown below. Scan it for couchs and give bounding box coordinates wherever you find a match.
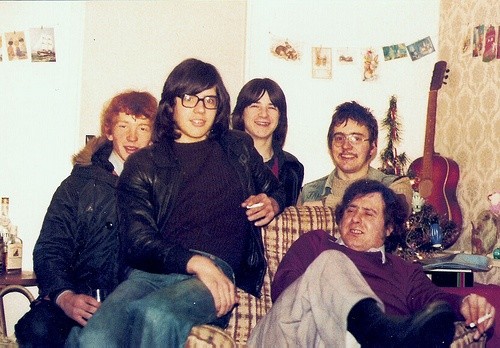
[184,205,489,348]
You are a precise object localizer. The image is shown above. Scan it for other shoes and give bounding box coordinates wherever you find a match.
[399,300,456,348]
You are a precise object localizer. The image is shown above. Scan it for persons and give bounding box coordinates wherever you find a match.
[295,100,400,206]
[76,58,287,348]
[230,78,305,207]
[15,90,159,348]
[245,178,496,348]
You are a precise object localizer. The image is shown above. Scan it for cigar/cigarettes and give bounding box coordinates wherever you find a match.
[246,202,264,208]
[469,312,492,327]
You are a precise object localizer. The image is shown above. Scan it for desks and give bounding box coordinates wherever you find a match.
[0,271,37,348]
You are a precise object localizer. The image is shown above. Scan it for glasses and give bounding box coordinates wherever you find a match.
[176,93,223,110]
[331,132,372,147]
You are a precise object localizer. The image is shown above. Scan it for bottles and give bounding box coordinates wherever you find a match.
[6,225,22,274]
[0,237,8,274]
[0,197,12,275]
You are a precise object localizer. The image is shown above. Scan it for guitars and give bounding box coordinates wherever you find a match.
[396,61,461,252]
